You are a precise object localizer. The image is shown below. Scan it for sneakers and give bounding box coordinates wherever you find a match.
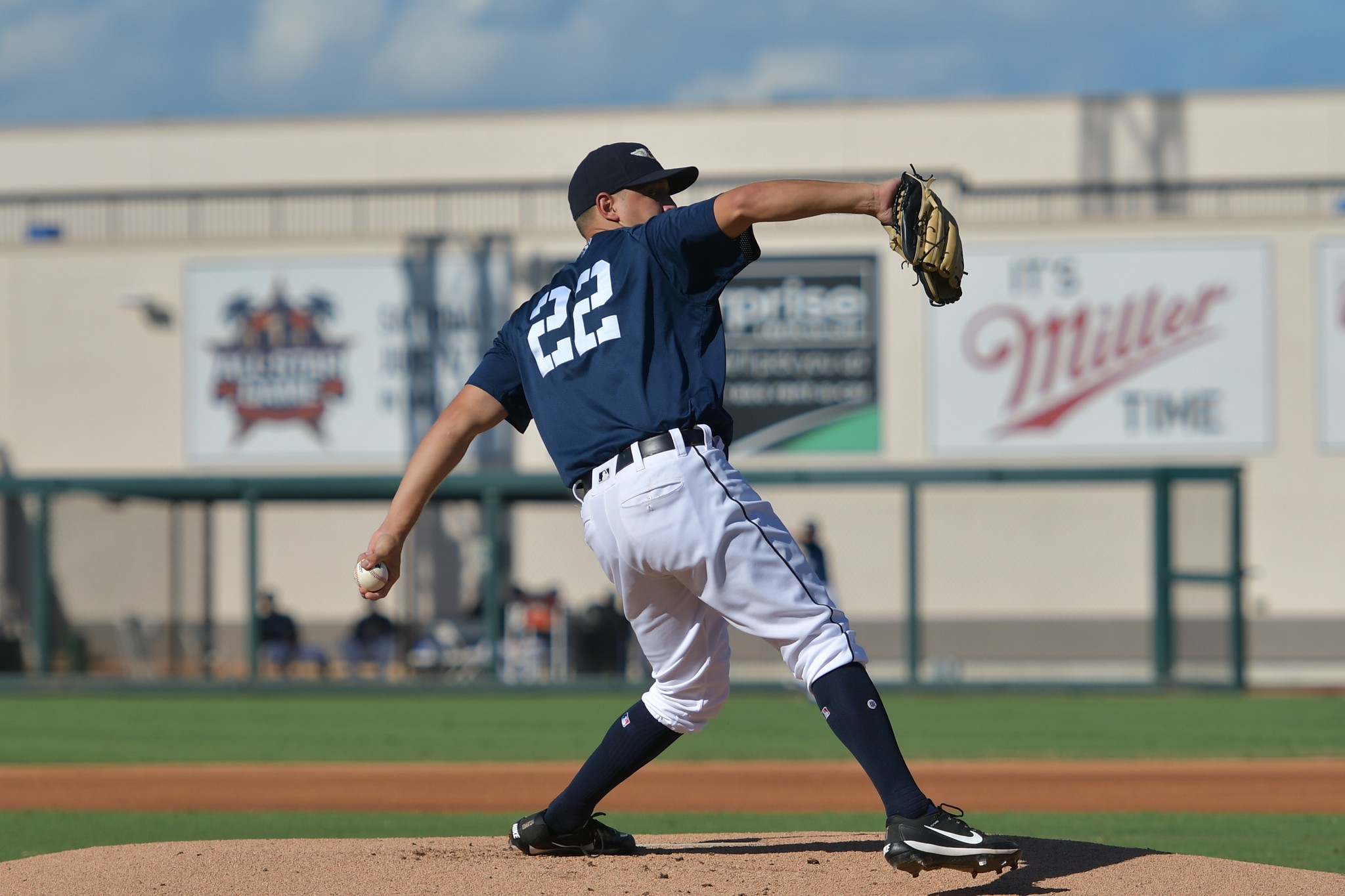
[884,798,1021,879]
[507,808,635,858]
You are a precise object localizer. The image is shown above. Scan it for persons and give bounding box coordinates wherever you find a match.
[470,585,633,679]
[779,517,830,592]
[248,590,325,678]
[356,143,1023,882]
[344,600,408,681]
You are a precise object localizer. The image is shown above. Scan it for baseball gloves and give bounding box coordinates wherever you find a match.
[880,171,966,304]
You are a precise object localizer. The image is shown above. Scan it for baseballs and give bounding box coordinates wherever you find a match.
[353,558,389,591]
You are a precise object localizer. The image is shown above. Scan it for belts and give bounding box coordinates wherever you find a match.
[581,430,728,494]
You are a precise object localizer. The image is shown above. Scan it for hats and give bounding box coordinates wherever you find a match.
[568,143,699,221]
